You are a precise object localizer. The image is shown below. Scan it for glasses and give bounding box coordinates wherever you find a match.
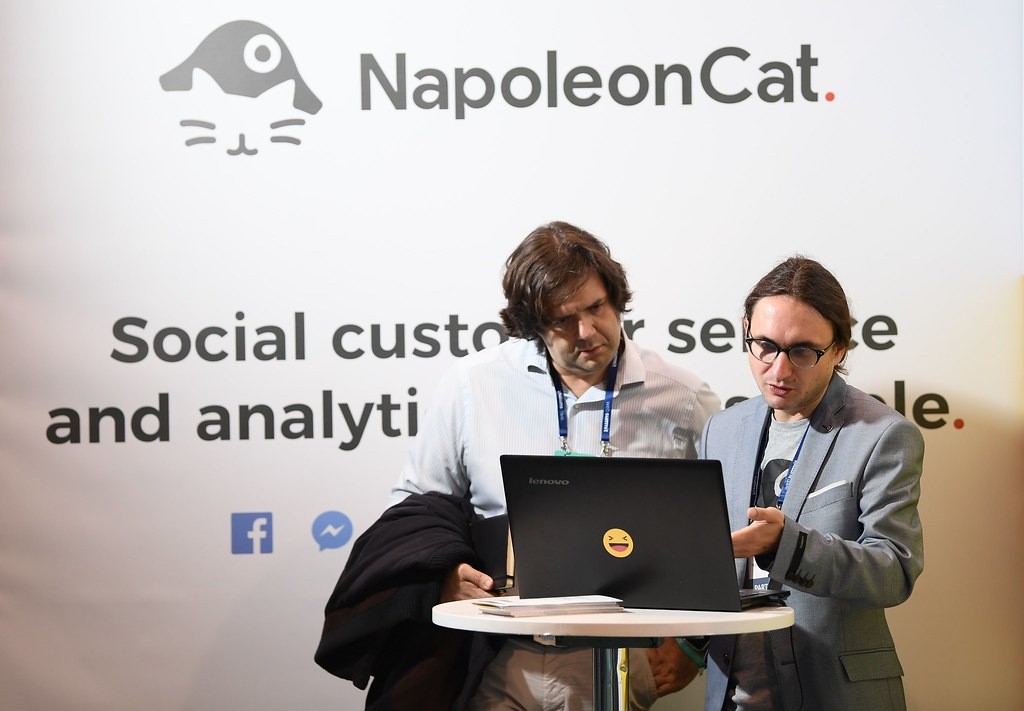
[745,321,837,369]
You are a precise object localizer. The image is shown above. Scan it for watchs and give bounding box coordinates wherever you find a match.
[683,636,713,652]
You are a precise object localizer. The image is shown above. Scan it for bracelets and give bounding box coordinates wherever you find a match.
[674,637,706,668]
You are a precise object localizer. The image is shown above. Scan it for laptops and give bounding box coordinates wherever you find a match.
[500,454,792,613]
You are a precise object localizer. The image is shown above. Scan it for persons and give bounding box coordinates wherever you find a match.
[698,257,925,710]
[391,221,721,711]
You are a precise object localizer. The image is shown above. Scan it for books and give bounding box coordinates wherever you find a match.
[469,595,625,617]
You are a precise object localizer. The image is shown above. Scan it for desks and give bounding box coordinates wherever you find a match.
[432,596,794,711]
